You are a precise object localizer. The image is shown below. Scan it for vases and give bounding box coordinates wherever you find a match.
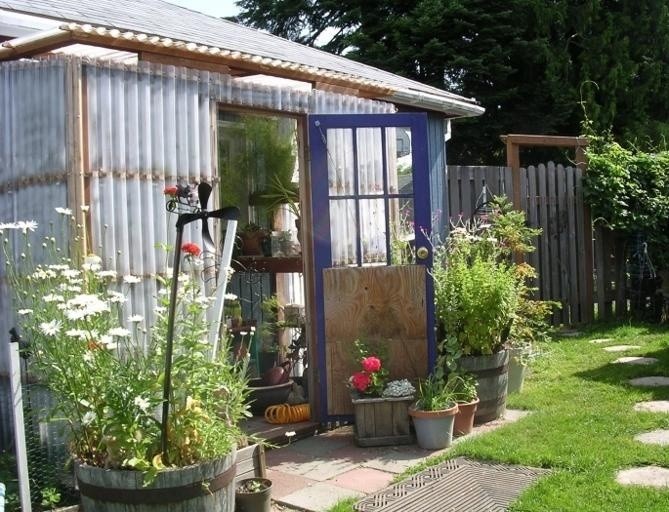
[346,385,417,448]
[63,434,240,512]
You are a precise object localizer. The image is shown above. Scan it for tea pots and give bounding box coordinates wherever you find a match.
[278,301,305,323]
[263,360,292,385]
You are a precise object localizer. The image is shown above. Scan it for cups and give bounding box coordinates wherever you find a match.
[262,241,272,257]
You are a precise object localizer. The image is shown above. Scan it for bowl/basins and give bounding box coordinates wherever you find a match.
[241,377,292,415]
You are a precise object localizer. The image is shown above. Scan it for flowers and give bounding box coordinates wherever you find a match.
[0,179,295,498]
[342,335,417,398]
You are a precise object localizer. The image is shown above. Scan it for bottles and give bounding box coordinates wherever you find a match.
[224,299,241,328]
[272,231,291,257]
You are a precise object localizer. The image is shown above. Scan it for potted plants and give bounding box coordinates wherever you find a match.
[237,220,267,256]
[441,370,482,436]
[232,474,275,512]
[425,191,566,426]
[402,369,460,450]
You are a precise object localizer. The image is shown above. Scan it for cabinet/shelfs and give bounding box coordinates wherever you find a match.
[222,186,307,330]
